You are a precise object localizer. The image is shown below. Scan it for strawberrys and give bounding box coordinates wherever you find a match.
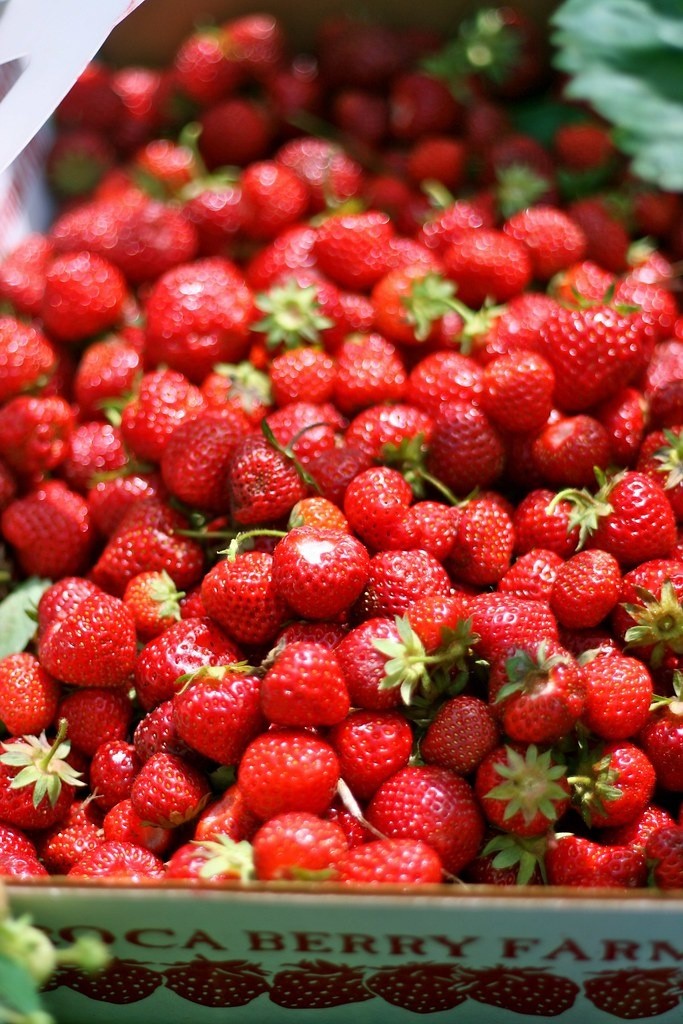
[0,0,683,884]
